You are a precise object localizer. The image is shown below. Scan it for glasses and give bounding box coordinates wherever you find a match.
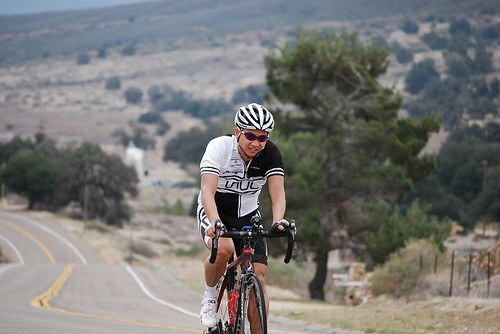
[240,131,270,143]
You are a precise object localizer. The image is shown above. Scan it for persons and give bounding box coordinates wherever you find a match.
[197,104,297,334]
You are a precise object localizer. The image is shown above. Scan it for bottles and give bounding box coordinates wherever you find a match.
[234,274,244,291]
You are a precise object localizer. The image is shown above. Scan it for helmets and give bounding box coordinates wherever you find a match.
[233,102,274,134]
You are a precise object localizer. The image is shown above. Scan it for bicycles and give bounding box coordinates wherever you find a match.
[209,217,298,333]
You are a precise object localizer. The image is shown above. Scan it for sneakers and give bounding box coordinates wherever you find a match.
[199,291,218,328]
